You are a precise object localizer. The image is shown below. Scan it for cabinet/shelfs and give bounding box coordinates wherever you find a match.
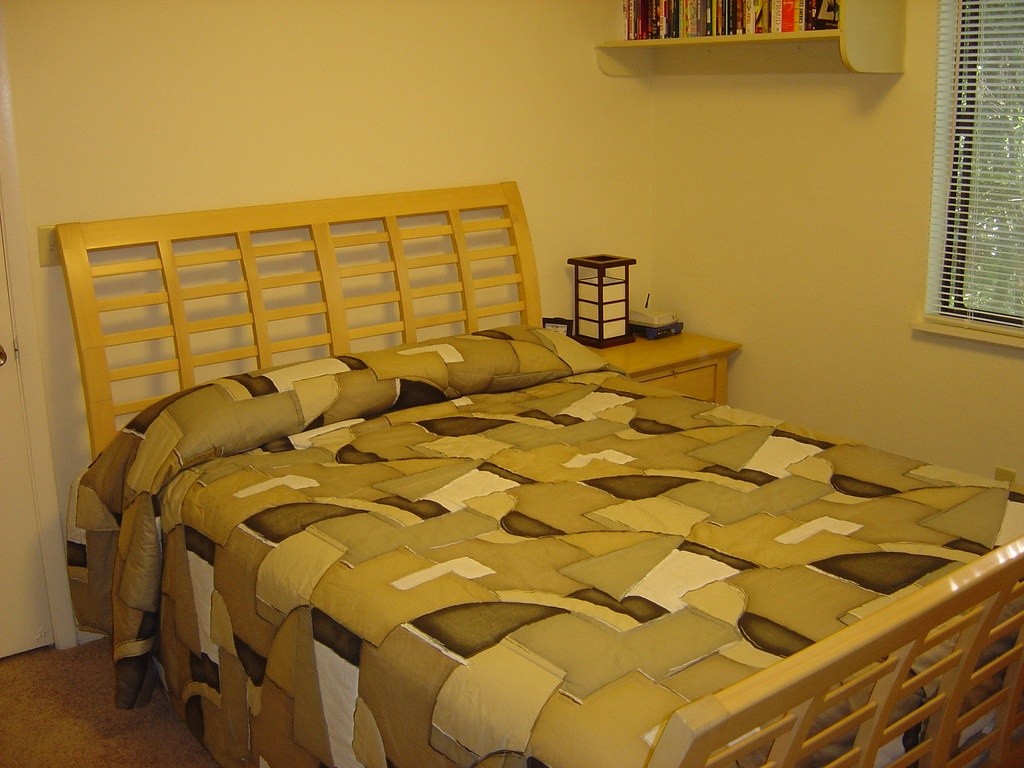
[590,0,905,77]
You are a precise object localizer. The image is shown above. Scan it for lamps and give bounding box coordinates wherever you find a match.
[567,253,637,350]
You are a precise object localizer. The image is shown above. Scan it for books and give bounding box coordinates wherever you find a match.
[622,0,840,41]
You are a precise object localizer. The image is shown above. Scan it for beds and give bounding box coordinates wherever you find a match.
[57,179,1024,768]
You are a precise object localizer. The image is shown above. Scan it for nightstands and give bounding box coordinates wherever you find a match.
[583,330,743,403]
